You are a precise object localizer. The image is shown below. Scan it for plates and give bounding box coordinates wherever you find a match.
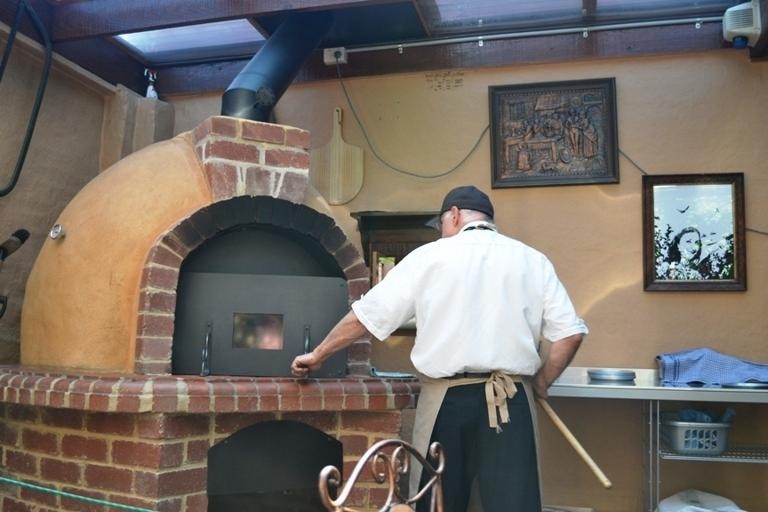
[587,369,636,380]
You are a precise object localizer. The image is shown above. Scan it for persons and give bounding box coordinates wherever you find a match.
[666,226,709,278]
[290,185,591,512]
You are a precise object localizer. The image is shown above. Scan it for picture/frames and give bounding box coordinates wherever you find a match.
[642,173,747,291]
[488,78,620,190]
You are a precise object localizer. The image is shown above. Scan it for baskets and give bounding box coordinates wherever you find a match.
[662,417,732,456]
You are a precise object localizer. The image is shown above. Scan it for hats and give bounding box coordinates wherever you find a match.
[425,185,494,229]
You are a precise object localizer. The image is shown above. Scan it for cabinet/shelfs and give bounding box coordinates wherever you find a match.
[644,398,767,512]
